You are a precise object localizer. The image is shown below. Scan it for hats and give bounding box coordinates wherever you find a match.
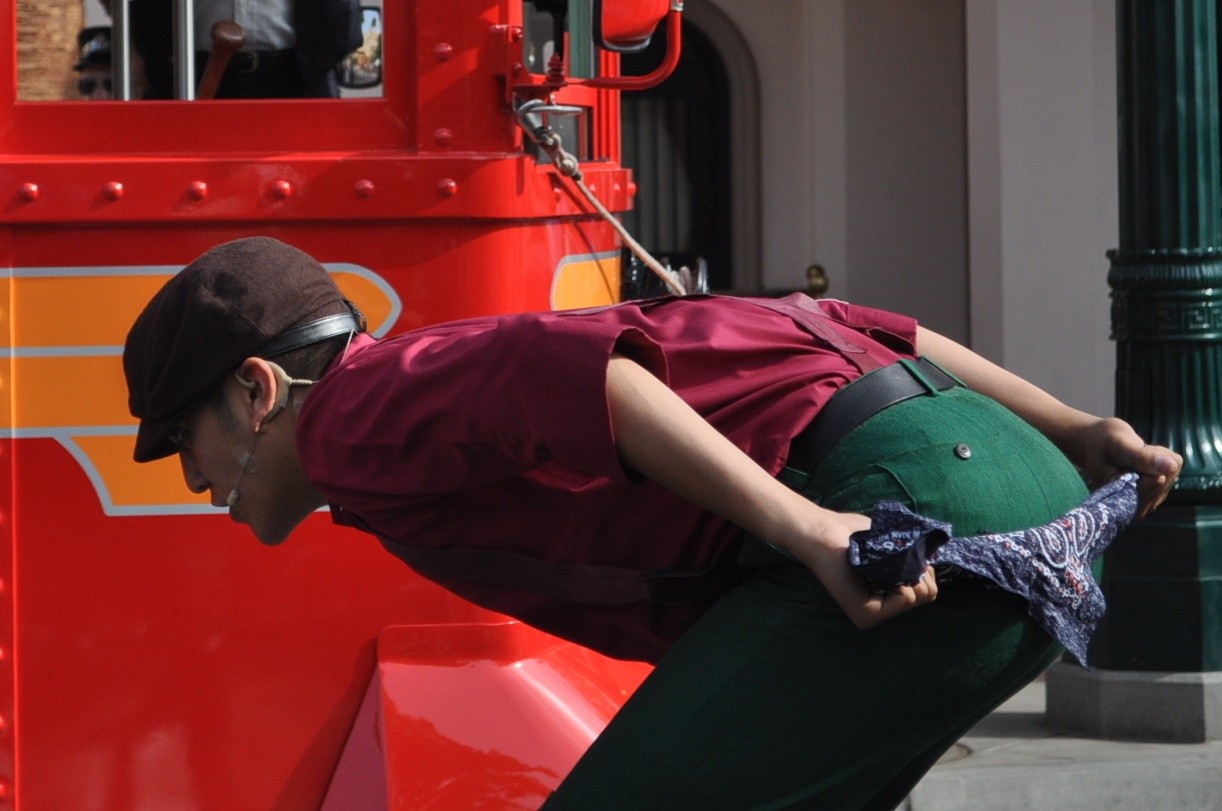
[122,235,359,463]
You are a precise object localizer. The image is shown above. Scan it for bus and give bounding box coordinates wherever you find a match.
[0,0,685,811]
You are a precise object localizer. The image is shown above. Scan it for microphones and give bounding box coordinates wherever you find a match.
[227,434,263,506]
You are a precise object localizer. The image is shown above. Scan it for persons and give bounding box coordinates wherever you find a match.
[98,0,364,100]
[121,235,1183,811]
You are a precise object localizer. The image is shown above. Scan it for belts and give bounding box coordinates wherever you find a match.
[790,361,956,477]
